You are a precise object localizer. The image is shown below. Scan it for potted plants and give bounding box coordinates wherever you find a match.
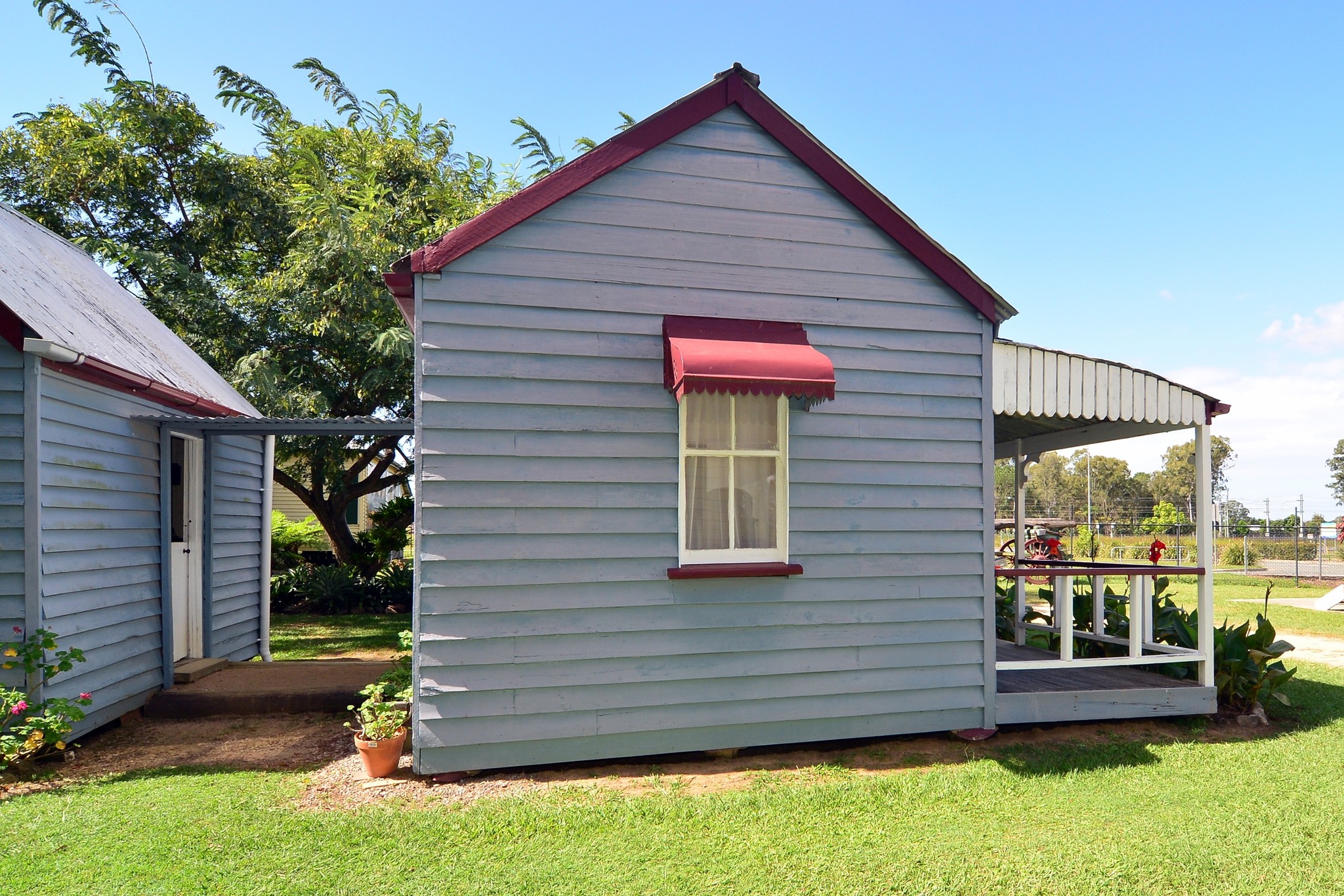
[344,680,407,779]
